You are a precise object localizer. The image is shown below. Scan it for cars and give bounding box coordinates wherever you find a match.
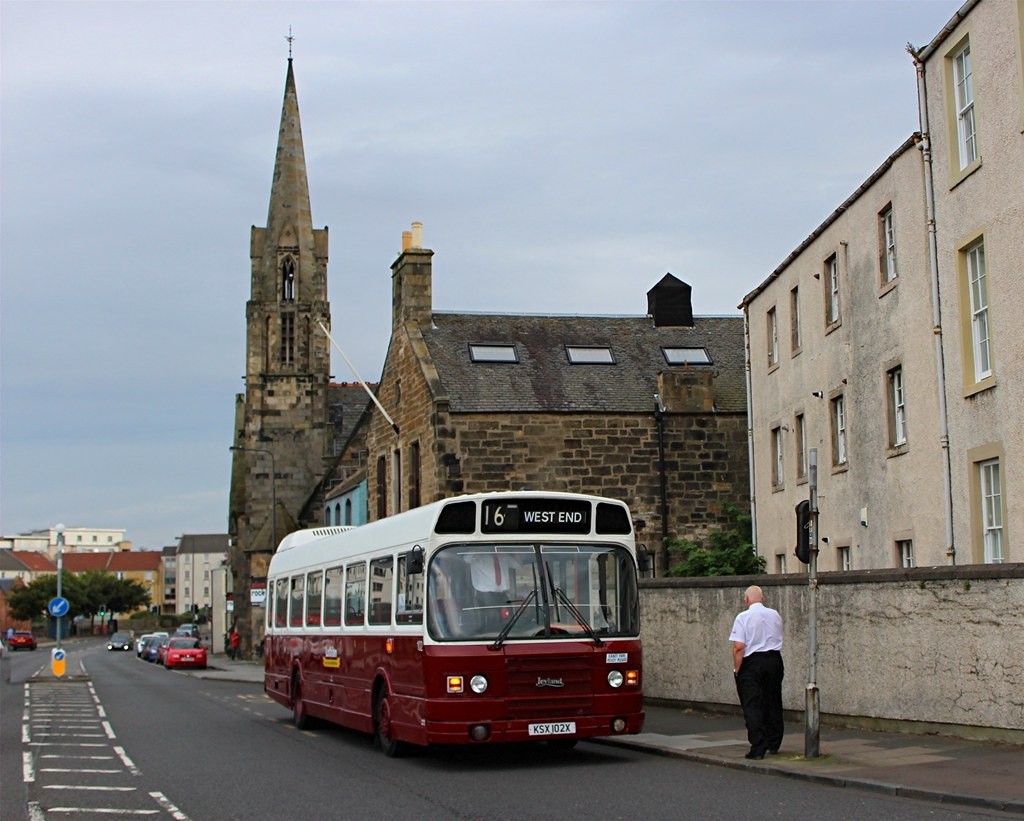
[107,632,134,652]
[170,623,200,636]
[137,632,170,662]
[172,631,190,638]
[155,636,209,669]
[8,631,37,651]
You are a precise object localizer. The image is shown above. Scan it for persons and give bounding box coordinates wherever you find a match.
[192,626,201,647]
[729,586,785,760]
[464,549,522,626]
[7,626,14,651]
[230,627,244,661]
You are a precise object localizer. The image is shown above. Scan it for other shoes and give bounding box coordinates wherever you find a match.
[745,746,766,760]
[769,749,778,754]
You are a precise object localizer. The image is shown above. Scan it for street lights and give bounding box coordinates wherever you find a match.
[174,537,197,622]
[52,522,66,649]
[229,446,276,557]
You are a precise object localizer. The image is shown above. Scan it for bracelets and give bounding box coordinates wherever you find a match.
[733,669,738,676]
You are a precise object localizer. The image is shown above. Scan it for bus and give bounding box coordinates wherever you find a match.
[265,490,655,761]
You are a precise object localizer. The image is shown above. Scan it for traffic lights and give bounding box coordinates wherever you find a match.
[100,605,105,616]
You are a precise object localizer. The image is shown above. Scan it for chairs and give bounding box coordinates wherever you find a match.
[269,602,392,627]
